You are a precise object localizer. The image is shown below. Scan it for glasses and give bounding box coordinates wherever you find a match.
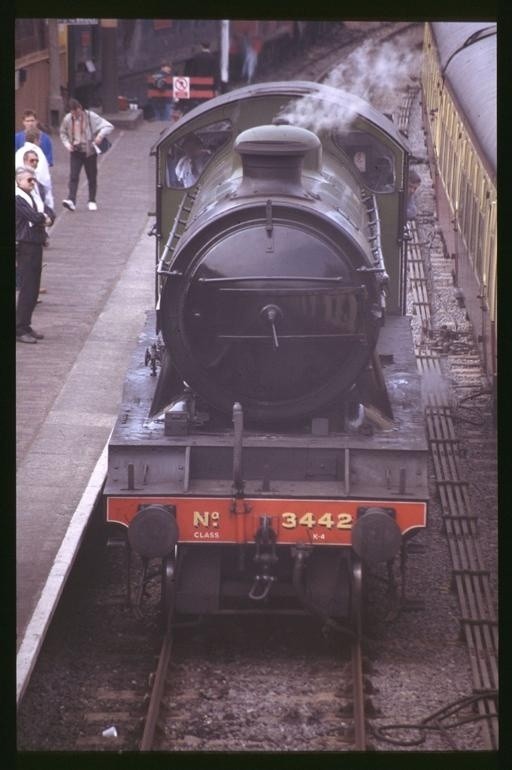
[26,177,38,183]
[29,158,39,163]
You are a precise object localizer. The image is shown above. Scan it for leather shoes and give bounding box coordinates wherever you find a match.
[15,330,45,344]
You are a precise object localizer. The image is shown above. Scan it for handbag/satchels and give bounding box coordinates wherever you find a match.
[35,287,48,304]
[92,137,111,154]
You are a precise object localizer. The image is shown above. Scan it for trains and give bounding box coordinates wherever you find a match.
[105,78,430,630]
[421,22,497,322]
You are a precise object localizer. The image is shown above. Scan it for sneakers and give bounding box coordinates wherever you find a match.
[87,201,98,211]
[62,199,75,211]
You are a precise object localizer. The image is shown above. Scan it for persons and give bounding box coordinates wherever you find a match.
[23,150,52,304]
[191,40,216,79]
[16,129,49,174]
[15,166,56,345]
[58,97,113,212]
[16,111,55,247]
